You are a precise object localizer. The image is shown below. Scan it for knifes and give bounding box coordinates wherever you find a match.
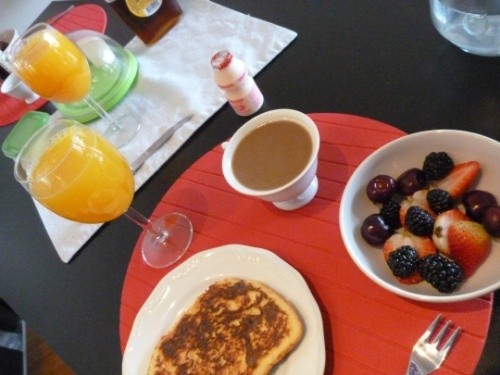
[131,114,194,173]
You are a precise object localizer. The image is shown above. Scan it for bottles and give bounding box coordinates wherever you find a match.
[211,50,265,118]
[104,0,185,46]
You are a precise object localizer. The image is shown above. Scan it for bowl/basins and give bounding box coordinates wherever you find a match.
[339,128,500,304]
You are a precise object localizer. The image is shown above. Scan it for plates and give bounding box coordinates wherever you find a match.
[121,244,326,375]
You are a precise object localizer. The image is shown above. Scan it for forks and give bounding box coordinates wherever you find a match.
[406,315,460,375]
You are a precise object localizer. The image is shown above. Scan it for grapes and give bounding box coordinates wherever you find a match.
[362,168,500,244]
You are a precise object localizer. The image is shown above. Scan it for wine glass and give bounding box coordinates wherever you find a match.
[6,21,142,149]
[12,117,193,269]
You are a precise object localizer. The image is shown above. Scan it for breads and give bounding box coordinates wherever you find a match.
[146,276,305,375]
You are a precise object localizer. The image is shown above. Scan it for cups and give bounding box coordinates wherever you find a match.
[1,73,42,104]
[428,0,500,59]
[221,108,319,211]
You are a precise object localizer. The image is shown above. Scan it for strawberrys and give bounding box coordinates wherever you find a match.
[447,220,492,278]
[429,161,480,201]
[431,209,475,256]
[399,189,439,228]
[383,227,439,284]
